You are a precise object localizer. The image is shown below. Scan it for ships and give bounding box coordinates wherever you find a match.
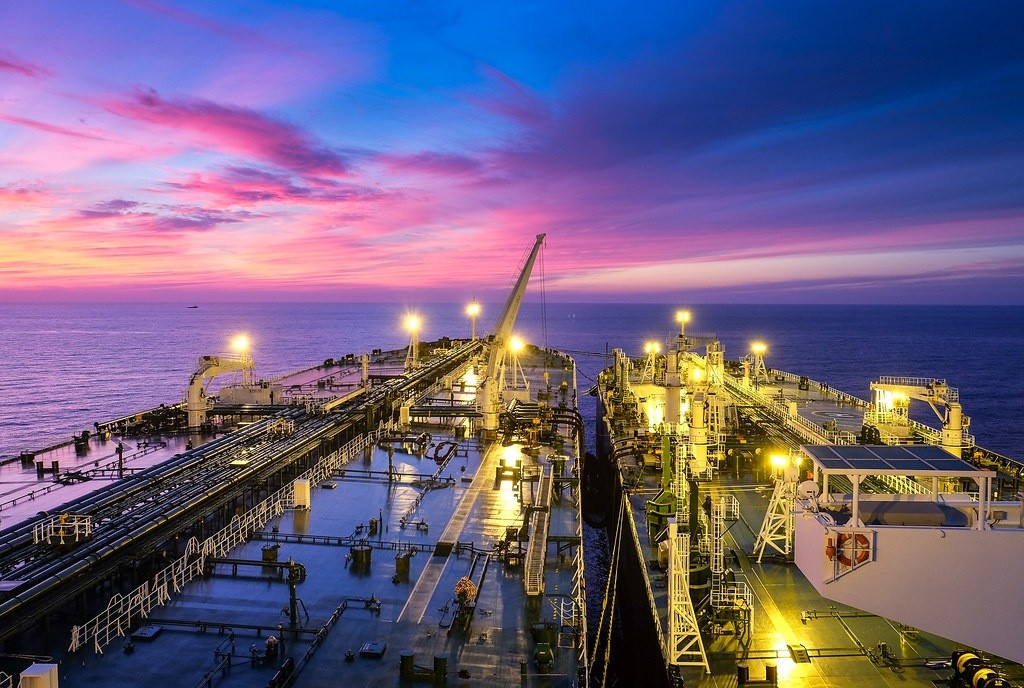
[590,310,1023,688]
[0,231,591,688]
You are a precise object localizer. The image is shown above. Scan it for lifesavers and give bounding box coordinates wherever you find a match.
[834,533,870,567]
